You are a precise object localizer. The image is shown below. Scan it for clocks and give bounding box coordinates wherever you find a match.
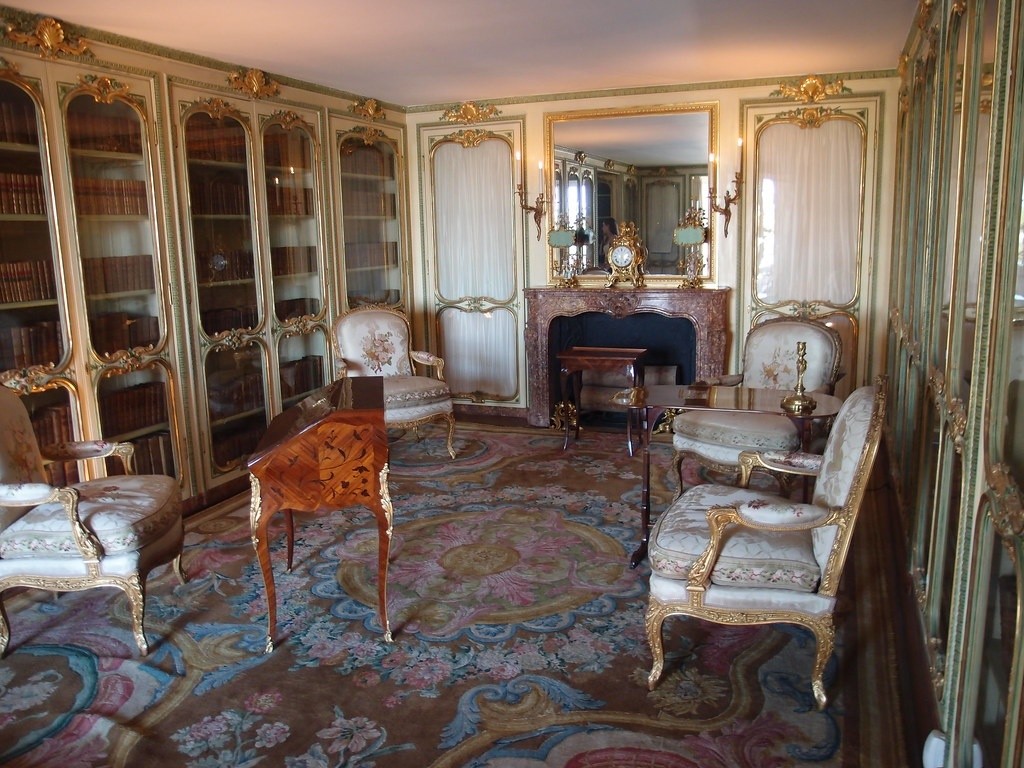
[605,220,649,290]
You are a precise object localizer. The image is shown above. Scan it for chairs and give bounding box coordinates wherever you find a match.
[670,315,843,500]
[644,374,889,711]
[326,305,458,460]
[0,386,188,656]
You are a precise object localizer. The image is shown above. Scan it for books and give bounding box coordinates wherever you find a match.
[0,103,400,487]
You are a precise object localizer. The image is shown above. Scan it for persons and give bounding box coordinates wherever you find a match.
[597,217,620,274]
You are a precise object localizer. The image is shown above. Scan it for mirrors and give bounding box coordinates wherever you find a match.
[543,100,719,286]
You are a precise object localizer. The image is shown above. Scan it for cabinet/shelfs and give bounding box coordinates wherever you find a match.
[0,46,409,601]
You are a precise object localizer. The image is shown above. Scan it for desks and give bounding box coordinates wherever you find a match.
[524,287,732,429]
[615,385,841,570]
[247,376,395,655]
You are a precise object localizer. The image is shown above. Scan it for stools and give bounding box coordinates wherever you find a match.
[554,346,648,458]
